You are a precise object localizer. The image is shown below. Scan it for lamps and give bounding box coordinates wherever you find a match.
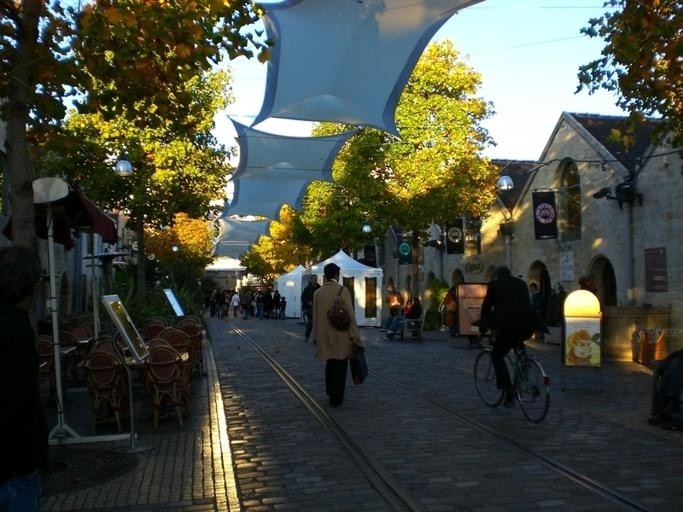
[116,160,133,176]
[497,176,514,190]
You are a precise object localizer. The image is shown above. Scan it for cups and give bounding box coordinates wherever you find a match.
[573,340,593,358]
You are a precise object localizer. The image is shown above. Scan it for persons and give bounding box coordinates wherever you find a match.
[479,263,552,407]
[302,274,322,342]
[312,263,360,407]
[529,283,545,339]
[0,247,48,512]
[379,296,422,342]
[209,285,286,321]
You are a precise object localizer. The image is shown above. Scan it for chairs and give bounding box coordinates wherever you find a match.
[33,314,207,433]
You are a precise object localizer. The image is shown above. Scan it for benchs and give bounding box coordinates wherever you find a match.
[394,308,427,341]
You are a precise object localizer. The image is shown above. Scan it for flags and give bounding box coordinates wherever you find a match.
[446,216,465,255]
[534,192,558,242]
[396,237,415,264]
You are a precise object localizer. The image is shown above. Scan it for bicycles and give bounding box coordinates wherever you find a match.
[474,326,550,423]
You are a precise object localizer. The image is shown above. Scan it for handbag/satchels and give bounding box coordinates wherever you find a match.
[327,296,350,332]
[349,343,367,384]
